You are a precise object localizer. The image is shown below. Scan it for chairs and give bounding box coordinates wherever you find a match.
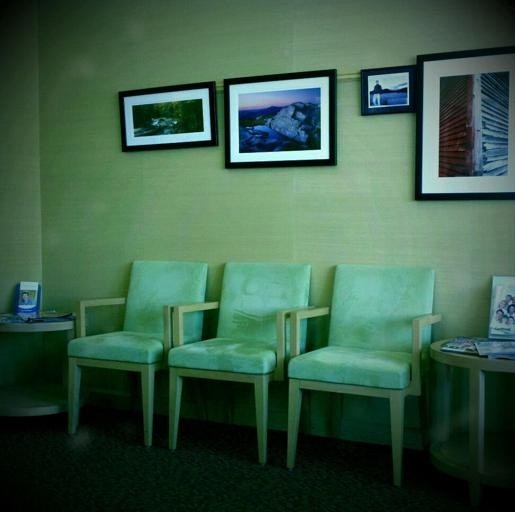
[287,265,442,487]
[167,263,310,467]
[68,260,208,447]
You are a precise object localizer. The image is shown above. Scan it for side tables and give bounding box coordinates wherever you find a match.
[431,340,515,508]
[0,321,85,417]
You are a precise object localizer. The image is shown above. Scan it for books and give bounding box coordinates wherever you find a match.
[440,335,515,360]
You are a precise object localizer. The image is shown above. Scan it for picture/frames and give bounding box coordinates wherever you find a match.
[360,65,416,116]
[223,69,337,169]
[415,46,515,201]
[119,81,219,152]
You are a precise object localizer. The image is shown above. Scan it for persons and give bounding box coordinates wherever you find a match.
[371,81,385,106]
[19,292,34,305]
[496,294,515,328]
